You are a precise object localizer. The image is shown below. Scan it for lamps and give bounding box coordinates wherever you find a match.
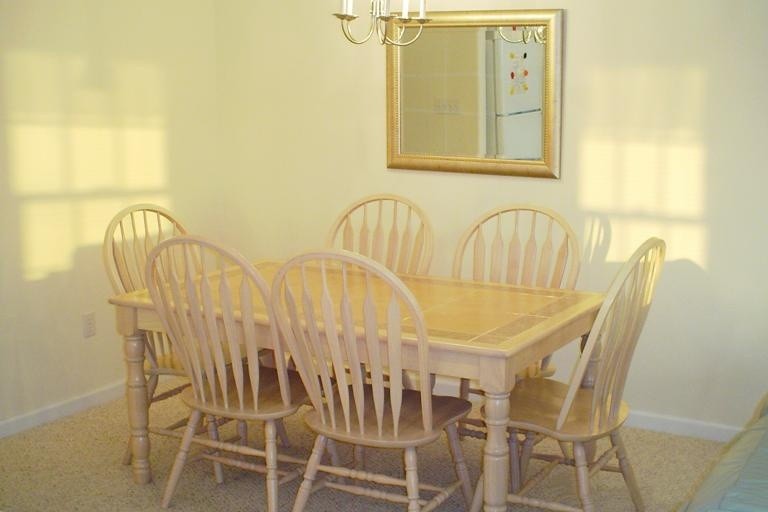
[332,0,432,46]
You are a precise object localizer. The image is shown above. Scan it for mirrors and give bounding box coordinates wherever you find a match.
[384,7,565,180]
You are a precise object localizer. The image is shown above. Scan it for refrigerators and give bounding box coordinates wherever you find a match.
[484,26,545,160]
